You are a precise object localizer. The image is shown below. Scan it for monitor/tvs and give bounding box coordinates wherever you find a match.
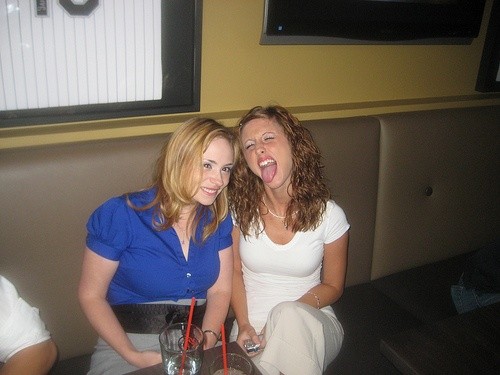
[259,0,486,45]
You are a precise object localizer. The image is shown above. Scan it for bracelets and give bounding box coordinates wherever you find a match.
[203,330,219,345]
[308,292,320,309]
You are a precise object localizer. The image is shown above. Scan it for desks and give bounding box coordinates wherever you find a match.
[380,302,500,375]
[123,342,261,375]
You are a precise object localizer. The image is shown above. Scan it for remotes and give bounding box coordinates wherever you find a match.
[244,339,261,353]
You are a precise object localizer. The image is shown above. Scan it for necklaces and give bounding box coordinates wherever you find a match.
[177,224,185,245]
[263,196,298,219]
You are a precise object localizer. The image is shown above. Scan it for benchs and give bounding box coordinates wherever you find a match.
[0,105,500,375]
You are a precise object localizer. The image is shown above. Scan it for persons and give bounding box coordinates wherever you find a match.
[227,105,352,375]
[0,274,58,375]
[77,116,235,375]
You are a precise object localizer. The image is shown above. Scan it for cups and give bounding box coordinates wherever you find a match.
[210,353,253,375]
[159,322,203,374]
